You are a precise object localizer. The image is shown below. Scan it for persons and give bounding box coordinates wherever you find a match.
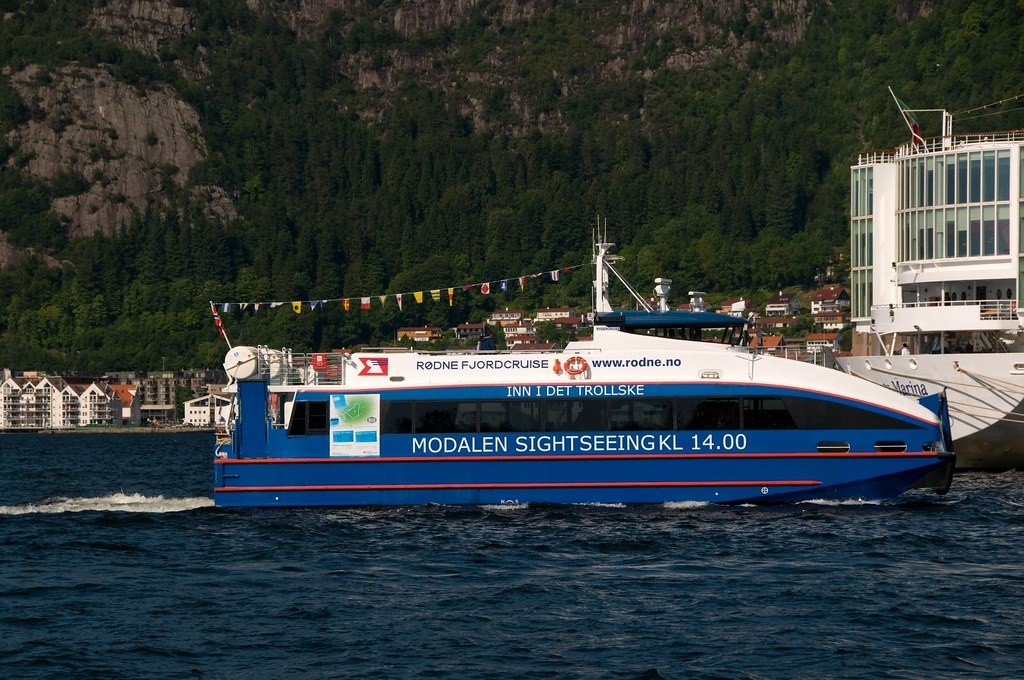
[477,330,497,355]
[965,341,972,353]
[945,292,951,306]
[901,343,910,355]
[947,340,953,352]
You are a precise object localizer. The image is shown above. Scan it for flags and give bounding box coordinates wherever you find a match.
[379,296,385,307]
[519,267,571,291]
[361,298,370,309]
[396,294,402,311]
[431,290,440,302]
[463,285,471,291]
[481,283,489,294]
[212,307,224,335]
[222,303,231,312]
[413,291,423,303]
[343,300,349,310]
[292,302,301,313]
[240,303,248,312]
[448,288,453,306]
[500,280,507,292]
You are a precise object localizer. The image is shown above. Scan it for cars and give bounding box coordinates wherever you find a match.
[182,423,194,428]
[154,421,168,428]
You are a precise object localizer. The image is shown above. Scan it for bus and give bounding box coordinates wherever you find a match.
[147,415,167,422]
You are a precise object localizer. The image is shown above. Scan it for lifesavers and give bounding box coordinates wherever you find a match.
[564,357,587,375]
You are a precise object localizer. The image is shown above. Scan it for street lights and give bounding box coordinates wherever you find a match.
[162,356,165,372]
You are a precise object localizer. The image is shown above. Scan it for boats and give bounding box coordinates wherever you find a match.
[212,242,957,508]
